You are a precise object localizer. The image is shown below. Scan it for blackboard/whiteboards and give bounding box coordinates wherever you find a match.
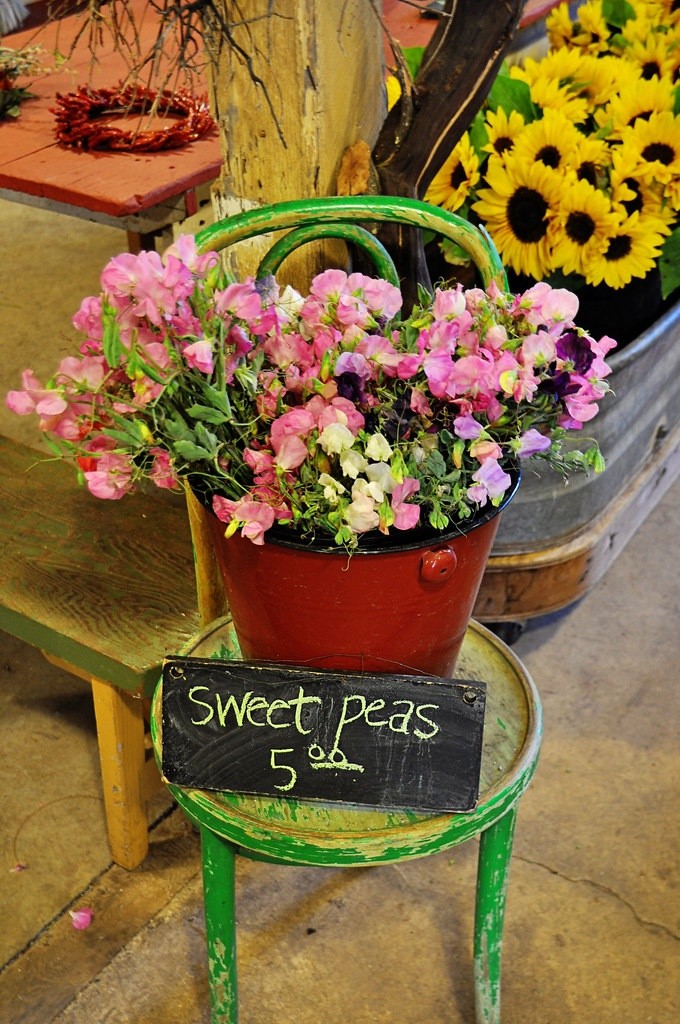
[158,656,486,814]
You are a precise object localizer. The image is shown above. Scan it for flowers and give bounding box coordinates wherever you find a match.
[4,230,619,571]
[382,1,680,301]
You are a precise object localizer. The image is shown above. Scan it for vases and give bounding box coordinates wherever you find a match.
[186,464,520,679]
[472,300,678,642]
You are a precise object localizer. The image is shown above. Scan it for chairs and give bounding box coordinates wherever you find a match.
[149,196,544,1023]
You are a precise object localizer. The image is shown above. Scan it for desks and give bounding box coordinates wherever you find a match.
[1,197,233,873]
[0,1,570,256]
